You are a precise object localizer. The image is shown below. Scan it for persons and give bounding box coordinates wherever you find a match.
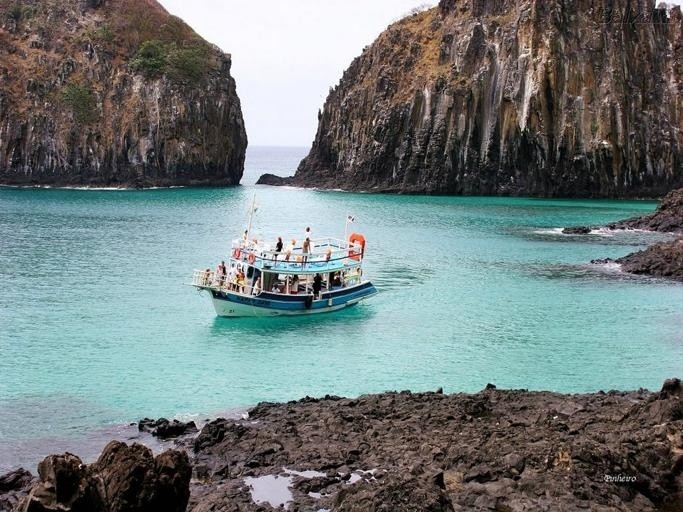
[252,277,261,296]
[271,283,280,294]
[202,261,227,287]
[312,273,323,300]
[271,237,296,264]
[232,230,257,265]
[301,227,312,266]
[329,271,341,291]
[291,274,299,294]
[228,263,246,293]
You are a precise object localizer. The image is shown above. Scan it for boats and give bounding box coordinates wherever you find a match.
[190,190,379,318]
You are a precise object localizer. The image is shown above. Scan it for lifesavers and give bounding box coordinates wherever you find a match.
[234,248,240,258]
[248,252,255,264]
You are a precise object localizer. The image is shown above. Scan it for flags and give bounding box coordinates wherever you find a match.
[346,215,355,224]
